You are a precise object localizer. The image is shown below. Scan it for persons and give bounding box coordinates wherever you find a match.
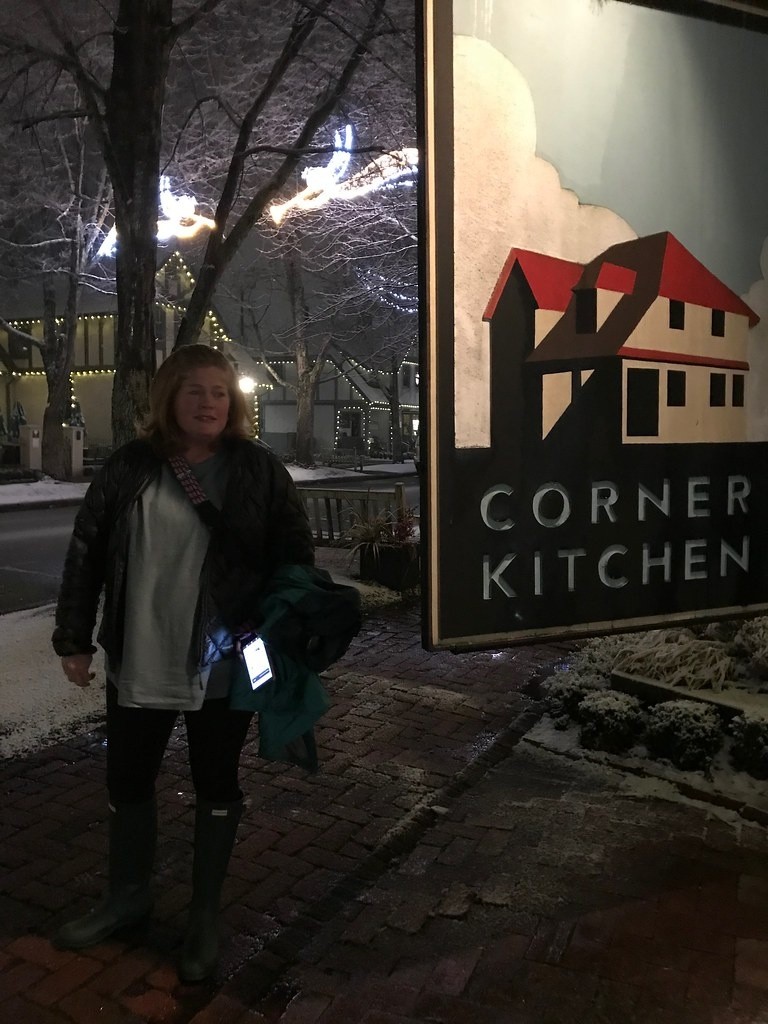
[50,344,315,980]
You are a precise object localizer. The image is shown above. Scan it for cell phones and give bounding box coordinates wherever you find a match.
[240,630,273,691]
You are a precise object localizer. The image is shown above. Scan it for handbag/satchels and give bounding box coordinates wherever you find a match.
[208,555,265,633]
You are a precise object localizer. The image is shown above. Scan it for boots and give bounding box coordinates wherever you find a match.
[179,788,245,984]
[52,797,158,948]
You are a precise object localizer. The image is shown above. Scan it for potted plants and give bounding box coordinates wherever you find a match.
[333,485,420,591]
